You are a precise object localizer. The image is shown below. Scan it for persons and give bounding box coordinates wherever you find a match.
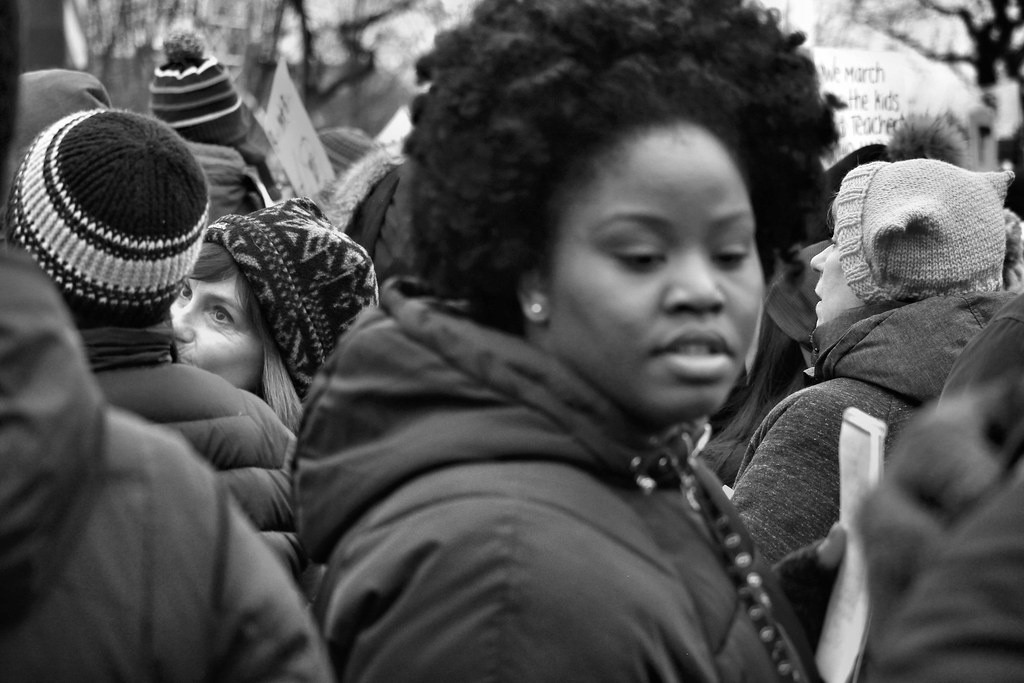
[731,159,1010,573]
[3,0,332,683]
[294,0,847,682]
[148,27,282,227]
[7,106,309,600]
[695,239,830,487]
[170,196,378,436]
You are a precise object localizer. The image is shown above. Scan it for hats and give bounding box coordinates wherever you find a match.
[4,109,208,330]
[826,111,971,201]
[203,196,379,403]
[834,159,1017,315]
[317,125,379,177]
[148,29,252,146]
